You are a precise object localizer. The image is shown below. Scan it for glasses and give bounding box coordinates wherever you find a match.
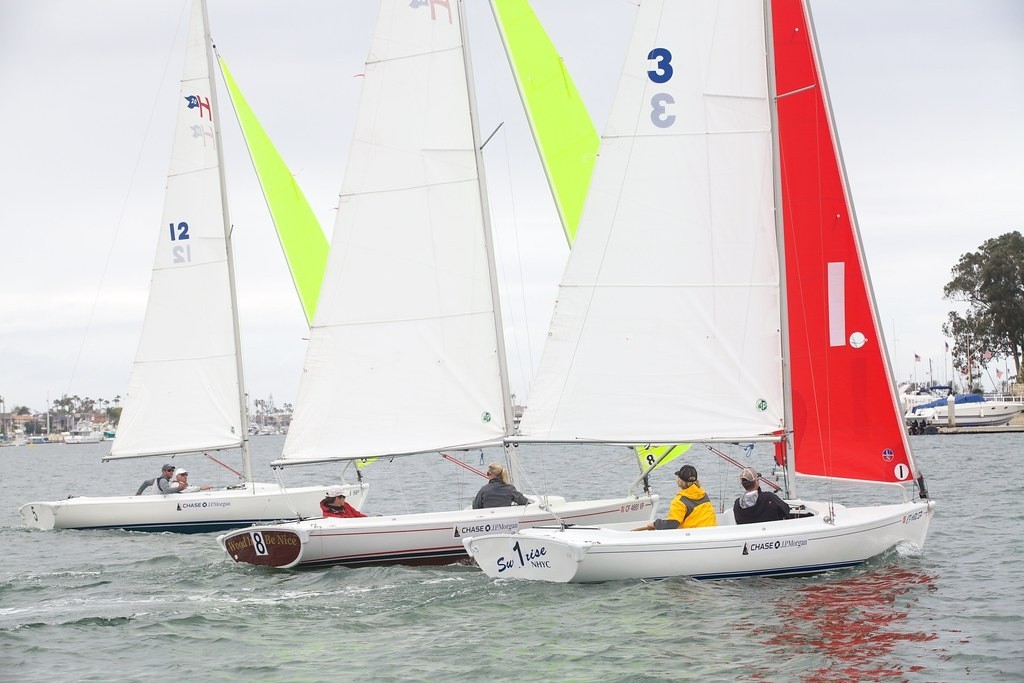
[486,472,493,476]
[338,495,345,499]
[165,469,174,472]
[179,473,188,476]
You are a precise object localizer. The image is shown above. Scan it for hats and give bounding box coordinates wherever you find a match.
[674,465,697,482]
[739,468,761,483]
[162,464,175,471]
[325,485,350,498]
[175,468,189,478]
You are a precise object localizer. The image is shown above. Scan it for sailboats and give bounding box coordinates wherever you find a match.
[19,0,371,535]
[0,396,17,446]
[216,0,658,570]
[463,0,935,582]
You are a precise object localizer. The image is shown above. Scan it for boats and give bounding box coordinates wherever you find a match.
[248,424,284,435]
[64,428,100,443]
[904,394,1024,427]
[25,437,52,443]
[904,385,953,400]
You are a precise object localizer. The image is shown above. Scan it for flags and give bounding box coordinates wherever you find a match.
[946,343,949,352]
[915,355,921,362]
[996,369,1003,378]
[1008,369,1010,374]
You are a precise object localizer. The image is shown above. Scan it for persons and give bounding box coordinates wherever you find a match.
[135,464,187,494]
[171,467,212,492]
[472,463,529,509]
[733,468,790,524]
[648,465,717,530]
[319,485,368,518]
